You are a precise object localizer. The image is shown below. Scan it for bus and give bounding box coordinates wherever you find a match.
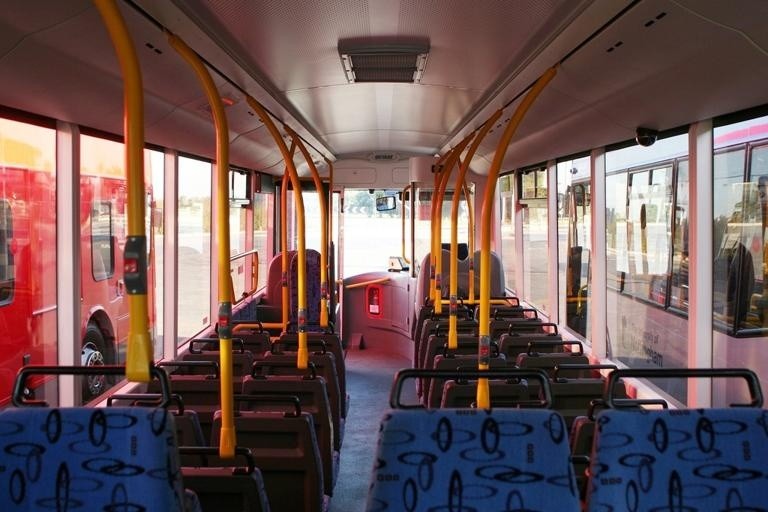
[0,165,156,407]
[562,135,768,410]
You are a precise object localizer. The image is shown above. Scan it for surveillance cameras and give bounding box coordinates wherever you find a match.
[369,189,374,193]
[634,127,657,146]
